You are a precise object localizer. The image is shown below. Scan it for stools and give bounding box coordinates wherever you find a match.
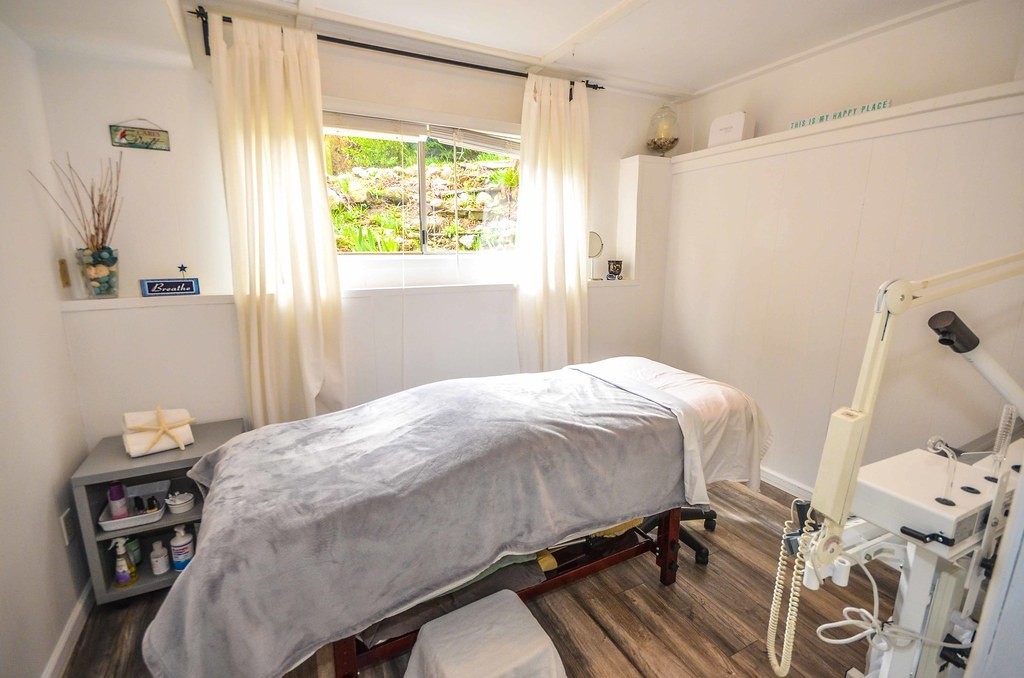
[403,588,569,678]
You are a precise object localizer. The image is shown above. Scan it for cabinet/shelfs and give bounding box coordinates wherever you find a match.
[70,417,246,604]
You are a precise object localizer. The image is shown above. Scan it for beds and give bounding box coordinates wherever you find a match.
[142,357,772,678]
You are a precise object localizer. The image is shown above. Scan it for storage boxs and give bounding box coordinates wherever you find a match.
[707,113,755,148]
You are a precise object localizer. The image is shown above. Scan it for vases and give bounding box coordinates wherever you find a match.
[77,250,118,300]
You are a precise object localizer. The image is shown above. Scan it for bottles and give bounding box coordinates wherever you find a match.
[107,481,128,519]
[149,540,171,575]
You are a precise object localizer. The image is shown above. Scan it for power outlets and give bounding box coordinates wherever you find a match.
[60,508,77,546]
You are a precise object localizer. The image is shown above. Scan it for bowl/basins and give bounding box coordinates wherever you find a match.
[167,493,195,514]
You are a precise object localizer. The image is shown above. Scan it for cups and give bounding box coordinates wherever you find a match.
[608,260,622,279]
